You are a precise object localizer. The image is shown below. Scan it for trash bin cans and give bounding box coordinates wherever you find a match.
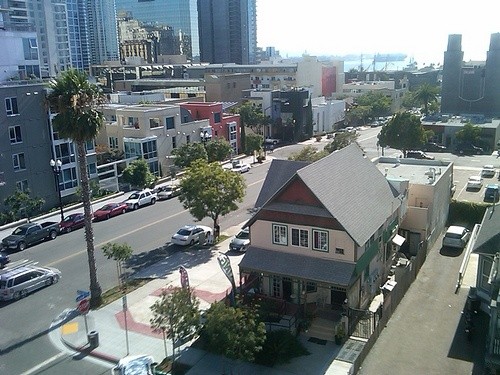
[88,330,99,348]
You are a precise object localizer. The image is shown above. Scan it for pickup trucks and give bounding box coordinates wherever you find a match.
[1,221,60,251]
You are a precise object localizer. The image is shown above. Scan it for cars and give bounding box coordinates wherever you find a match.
[58,212,85,233]
[483,183,500,203]
[93,203,128,221]
[466,176,484,190]
[482,164,497,178]
[230,163,251,174]
[229,227,251,253]
[264,135,281,145]
[171,225,213,246]
[335,129,349,137]
[345,126,357,133]
[370,116,389,128]
[158,185,183,200]
[442,225,472,249]
[0,241,11,269]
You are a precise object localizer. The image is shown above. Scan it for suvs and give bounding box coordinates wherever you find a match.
[122,190,159,210]
[407,150,436,160]
[0,265,60,303]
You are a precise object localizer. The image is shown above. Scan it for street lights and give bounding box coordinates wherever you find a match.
[263,103,278,149]
[50,158,64,221]
